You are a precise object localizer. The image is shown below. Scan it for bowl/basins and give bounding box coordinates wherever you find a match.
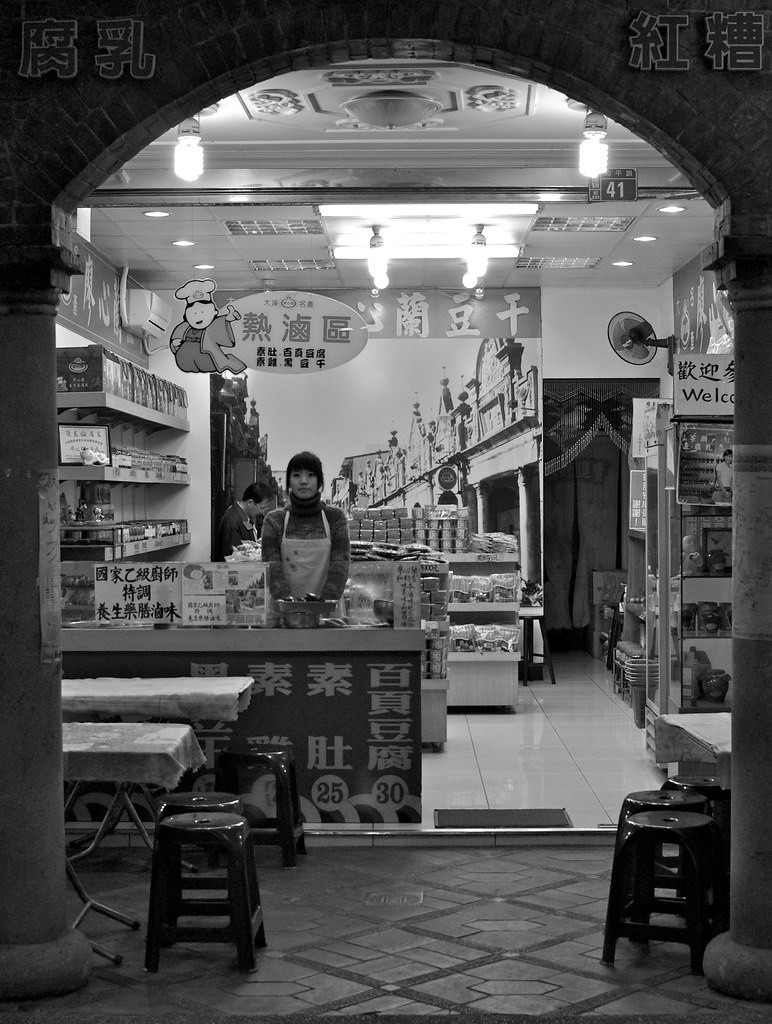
[373,599,395,624]
[279,599,341,628]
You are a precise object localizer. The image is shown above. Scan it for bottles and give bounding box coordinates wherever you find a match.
[703,609,720,633]
[707,550,727,575]
[702,669,728,702]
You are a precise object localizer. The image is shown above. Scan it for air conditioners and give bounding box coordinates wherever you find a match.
[123,289,172,339]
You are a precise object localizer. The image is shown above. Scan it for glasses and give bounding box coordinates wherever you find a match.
[255,502,268,514]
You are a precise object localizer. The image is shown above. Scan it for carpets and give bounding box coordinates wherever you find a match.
[433,808,573,829]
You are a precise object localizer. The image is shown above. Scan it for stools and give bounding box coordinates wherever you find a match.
[209,744,306,871]
[518,616,556,687]
[605,605,623,675]
[598,772,734,976]
[144,812,268,974]
[152,792,258,947]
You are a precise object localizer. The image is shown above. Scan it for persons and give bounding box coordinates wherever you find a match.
[261,451,351,628]
[714,449,733,498]
[218,482,271,562]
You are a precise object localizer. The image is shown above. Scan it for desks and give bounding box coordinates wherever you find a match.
[654,712,732,938]
[59,721,207,964]
[61,675,256,874]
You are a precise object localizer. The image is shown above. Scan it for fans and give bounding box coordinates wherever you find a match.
[608,312,675,376]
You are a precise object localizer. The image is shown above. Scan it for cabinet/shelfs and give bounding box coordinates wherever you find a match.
[57,389,192,563]
[434,551,523,709]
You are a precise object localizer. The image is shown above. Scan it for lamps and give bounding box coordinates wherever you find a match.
[339,89,442,130]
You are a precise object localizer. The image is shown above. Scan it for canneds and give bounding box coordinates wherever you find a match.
[65,446,187,542]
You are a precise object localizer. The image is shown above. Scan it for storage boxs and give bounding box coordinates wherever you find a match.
[589,568,629,660]
[55,344,190,544]
